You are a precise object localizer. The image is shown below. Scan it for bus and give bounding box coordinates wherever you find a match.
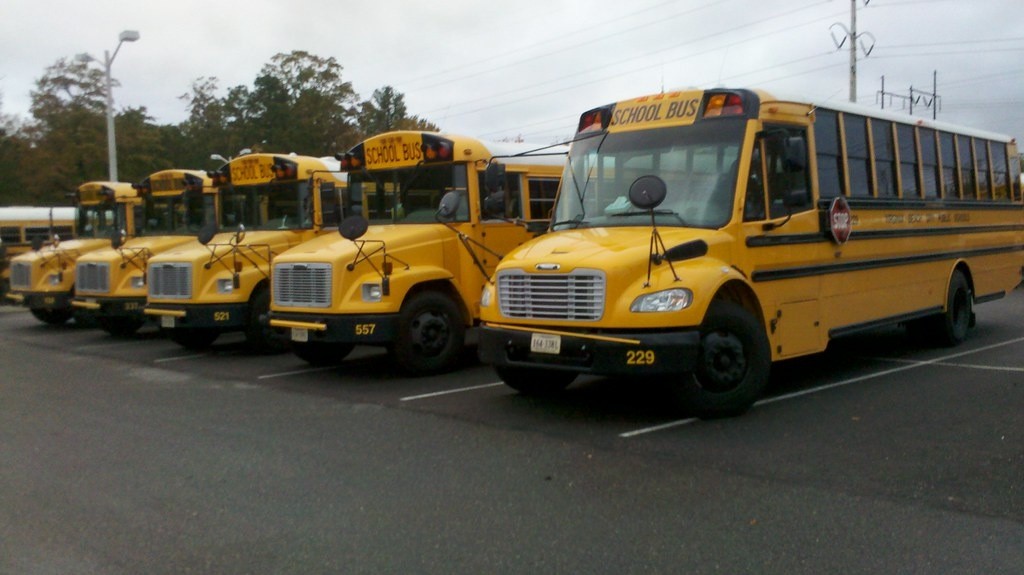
[268,130,566,378]
[1,205,76,304]
[479,86,1022,414]
[74,166,217,332]
[144,153,343,347]
[6,177,141,327]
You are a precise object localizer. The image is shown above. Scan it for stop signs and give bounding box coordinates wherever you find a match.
[828,196,853,245]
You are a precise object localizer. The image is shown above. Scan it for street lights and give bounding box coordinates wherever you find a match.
[104,28,142,179]
